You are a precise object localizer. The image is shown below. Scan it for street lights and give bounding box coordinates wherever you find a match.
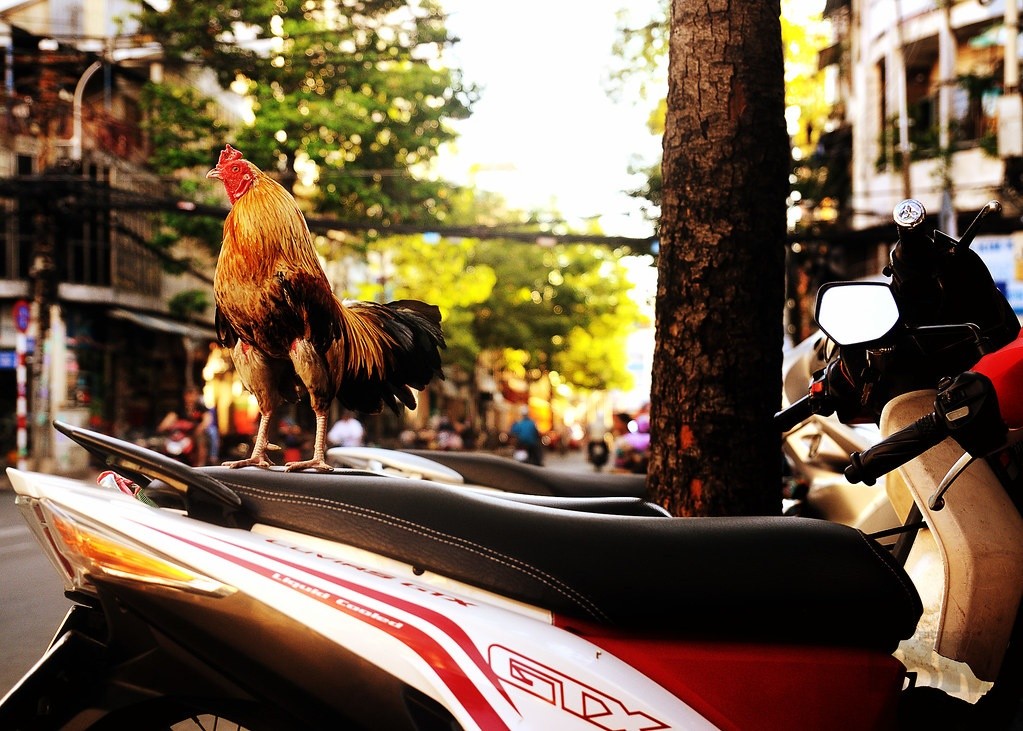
[70,44,165,162]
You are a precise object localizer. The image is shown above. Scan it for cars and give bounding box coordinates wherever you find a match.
[618,410,654,472]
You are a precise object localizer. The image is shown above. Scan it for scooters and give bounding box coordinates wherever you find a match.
[1,198,1023,730]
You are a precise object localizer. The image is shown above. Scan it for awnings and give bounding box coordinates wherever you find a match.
[117,310,226,343]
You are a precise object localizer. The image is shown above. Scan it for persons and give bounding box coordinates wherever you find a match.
[326,410,364,447]
[588,412,608,455]
[510,407,541,460]
[189,402,219,466]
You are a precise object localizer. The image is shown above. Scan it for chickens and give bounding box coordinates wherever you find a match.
[207,144,449,475]
